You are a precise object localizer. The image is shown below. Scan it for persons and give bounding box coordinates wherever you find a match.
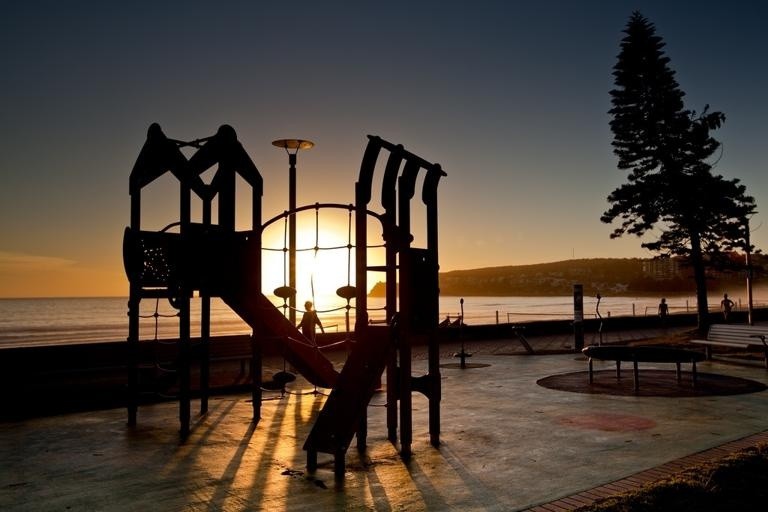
[658,298,669,335]
[720,293,734,325]
[295,300,325,346]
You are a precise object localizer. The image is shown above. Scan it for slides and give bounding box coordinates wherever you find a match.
[222,291,341,387]
[303,327,389,458]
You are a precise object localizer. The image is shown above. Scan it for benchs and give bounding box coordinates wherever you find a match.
[154,333,254,380]
[687,323,767,368]
